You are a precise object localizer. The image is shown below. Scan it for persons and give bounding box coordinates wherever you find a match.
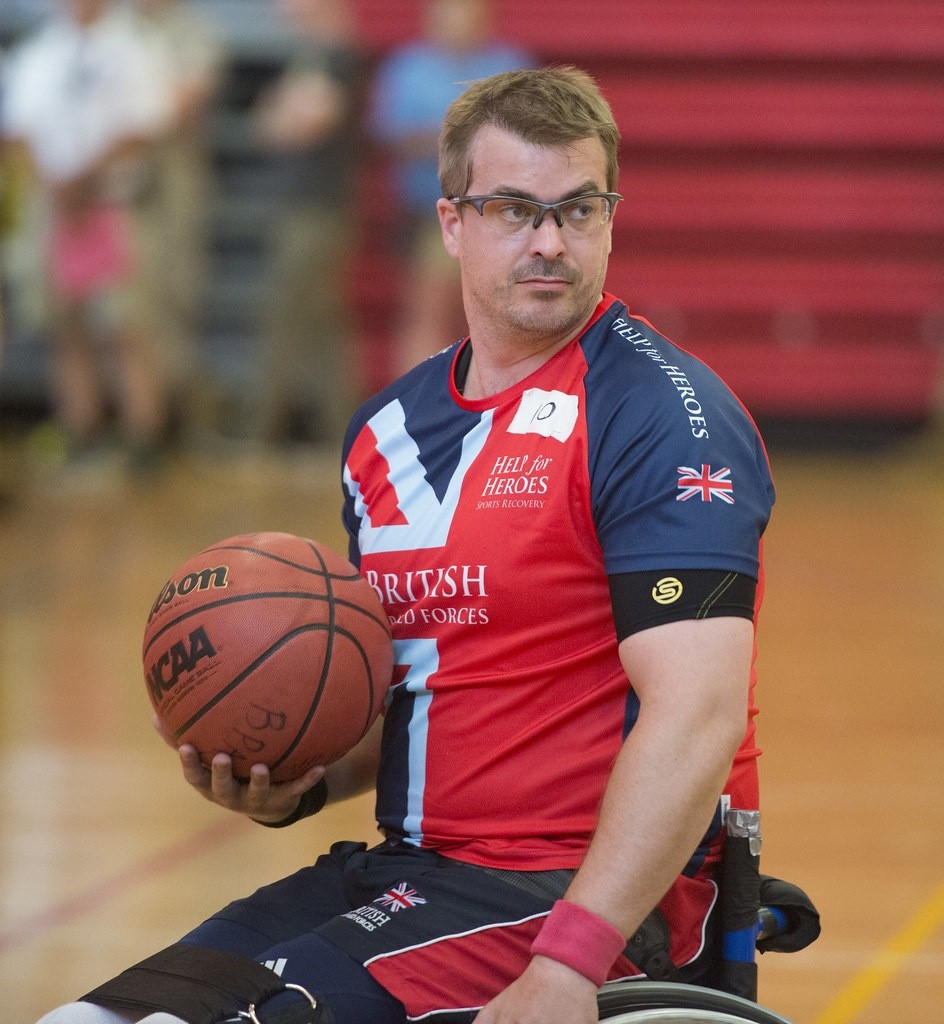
[252,1,367,448]
[38,69,777,1024]
[368,0,535,373]
[0,1,229,487]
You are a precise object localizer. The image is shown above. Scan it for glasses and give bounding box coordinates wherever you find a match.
[449,192,624,237]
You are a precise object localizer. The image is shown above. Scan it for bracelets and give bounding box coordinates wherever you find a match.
[249,777,329,829]
[531,899,626,987]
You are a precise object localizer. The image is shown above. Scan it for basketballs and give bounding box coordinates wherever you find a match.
[141,527,398,787]
